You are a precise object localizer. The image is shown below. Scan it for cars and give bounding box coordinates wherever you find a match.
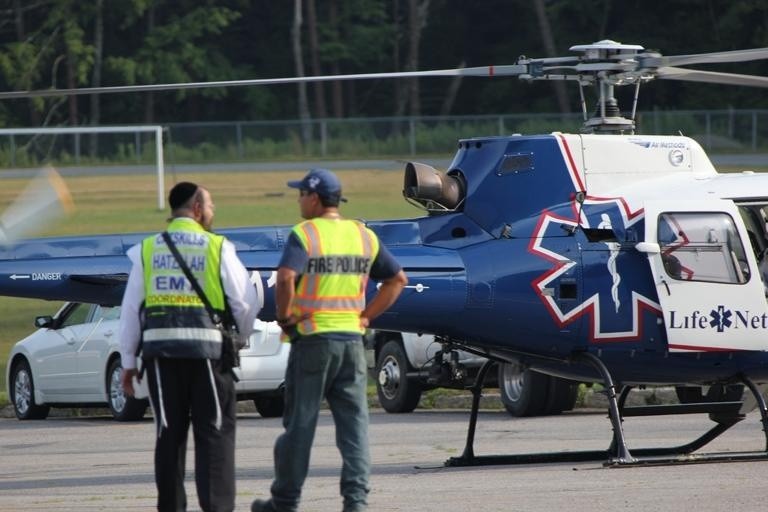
[370,327,726,416]
[7,300,292,422]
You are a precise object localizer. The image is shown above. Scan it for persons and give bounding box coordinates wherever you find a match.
[250,167,409,512]
[117,181,260,512]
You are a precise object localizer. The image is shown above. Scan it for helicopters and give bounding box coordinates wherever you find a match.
[0,37,768,467]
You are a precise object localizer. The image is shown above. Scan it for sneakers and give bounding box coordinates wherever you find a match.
[340,499,366,512]
[250,497,300,511]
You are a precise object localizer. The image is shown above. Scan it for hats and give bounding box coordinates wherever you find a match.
[287,169,341,196]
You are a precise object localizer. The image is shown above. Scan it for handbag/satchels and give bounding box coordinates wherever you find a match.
[222,322,241,367]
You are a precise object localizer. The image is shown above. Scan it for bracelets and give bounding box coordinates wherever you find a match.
[277,316,290,324]
